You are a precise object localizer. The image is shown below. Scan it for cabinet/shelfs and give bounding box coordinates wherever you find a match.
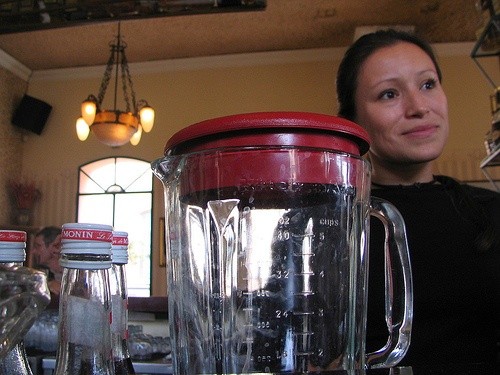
[0,224,45,269]
[469,16,500,192]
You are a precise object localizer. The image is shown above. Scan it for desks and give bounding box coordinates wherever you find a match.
[24,347,175,375]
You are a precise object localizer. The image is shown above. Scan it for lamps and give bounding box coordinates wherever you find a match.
[76,20,154,146]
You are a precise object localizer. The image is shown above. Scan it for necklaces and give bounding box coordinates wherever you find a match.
[370,173,437,187]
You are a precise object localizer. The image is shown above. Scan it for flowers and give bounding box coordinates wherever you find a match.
[12,183,41,212]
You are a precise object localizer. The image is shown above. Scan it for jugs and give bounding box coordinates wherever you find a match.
[150,112,413,375]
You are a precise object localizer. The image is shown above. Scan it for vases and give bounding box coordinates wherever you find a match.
[16,208,32,225]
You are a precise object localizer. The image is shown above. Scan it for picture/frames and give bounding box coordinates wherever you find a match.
[159,218,168,266]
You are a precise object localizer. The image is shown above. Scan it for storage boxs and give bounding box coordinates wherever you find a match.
[475,0,500,53]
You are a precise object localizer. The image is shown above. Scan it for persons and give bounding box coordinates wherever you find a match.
[336,29,500,375]
[33,226,64,269]
[32,235,63,310]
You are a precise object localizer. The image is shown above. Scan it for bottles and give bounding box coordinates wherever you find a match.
[0,231,34,375]
[112,232,137,375]
[54,223,117,375]
